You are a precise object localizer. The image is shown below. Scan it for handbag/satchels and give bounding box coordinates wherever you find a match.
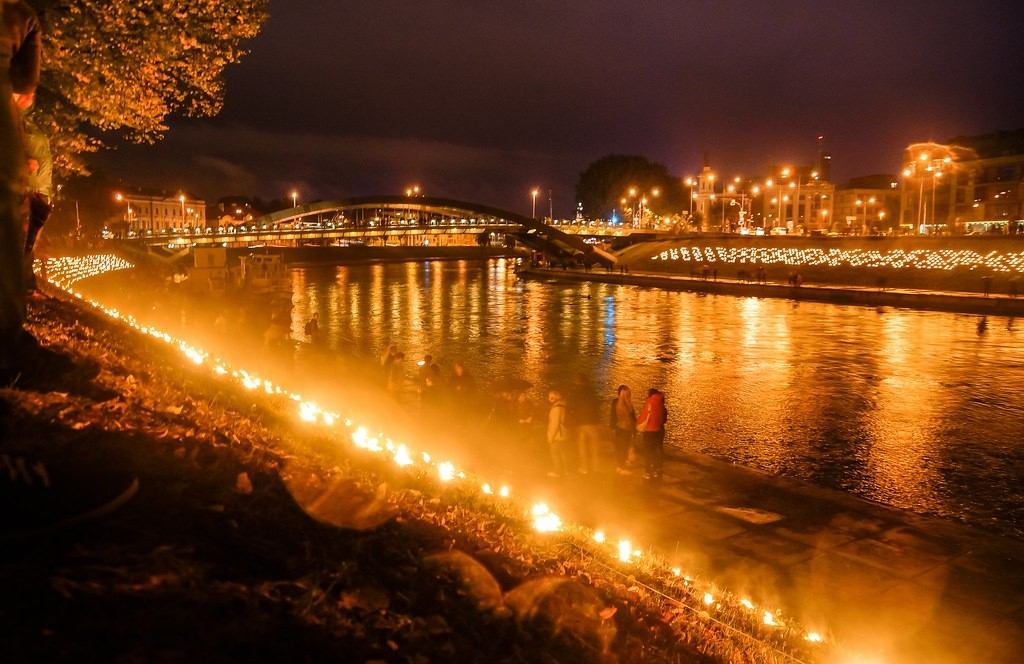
[636,403,651,432]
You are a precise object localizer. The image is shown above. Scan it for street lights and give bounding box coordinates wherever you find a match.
[293,192,296,208]
[778,168,789,228]
[856,198,876,225]
[179,195,186,231]
[115,194,131,223]
[622,186,660,230]
[685,178,698,218]
[903,150,951,238]
[532,188,538,220]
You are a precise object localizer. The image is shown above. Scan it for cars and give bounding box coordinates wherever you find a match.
[770,227,787,235]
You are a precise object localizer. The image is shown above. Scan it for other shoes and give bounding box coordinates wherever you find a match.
[643,472,651,479]
[547,471,560,477]
[577,467,588,474]
[615,467,633,475]
[653,471,659,478]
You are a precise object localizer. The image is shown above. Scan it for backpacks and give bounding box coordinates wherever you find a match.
[554,404,577,429]
[305,320,314,335]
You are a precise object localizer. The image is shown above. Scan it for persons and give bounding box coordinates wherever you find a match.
[0,0,70,369]
[610,384,636,476]
[530,257,630,274]
[690,264,717,281]
[1009,278,1018,297]
[981,276,991,297]
[179,297,319,353]
[635,388,667,478]
[544,385,599,476]
[737,266,766,284]
[382,345,535,450]
[988,221,1024,235]
[788,270,801,286]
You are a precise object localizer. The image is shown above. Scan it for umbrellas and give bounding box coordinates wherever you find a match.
[484,374,533,416]
